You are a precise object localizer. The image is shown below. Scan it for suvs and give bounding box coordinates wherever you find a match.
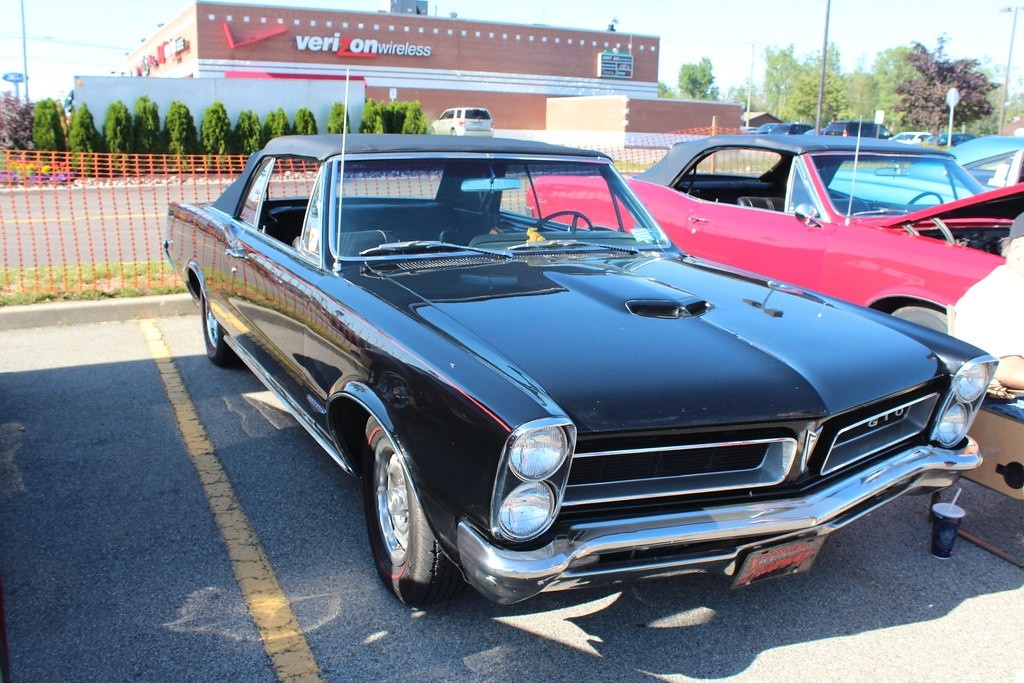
[429,107,494,137]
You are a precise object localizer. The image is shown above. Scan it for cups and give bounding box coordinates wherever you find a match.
[932,502,967,560]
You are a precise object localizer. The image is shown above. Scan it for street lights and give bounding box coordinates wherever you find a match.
[999,7,1023,135]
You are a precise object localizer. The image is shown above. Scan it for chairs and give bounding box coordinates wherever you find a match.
[441,223,499,247]
[339,228,399,256]
[737,196,785,212]
[831,197,866,215]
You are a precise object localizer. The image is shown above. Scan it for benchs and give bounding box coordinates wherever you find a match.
[262,201,452,241]
[680,182,783,203]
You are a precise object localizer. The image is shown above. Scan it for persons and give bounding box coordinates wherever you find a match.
[954,210,1024,390]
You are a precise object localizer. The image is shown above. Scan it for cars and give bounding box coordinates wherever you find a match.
[828,132,1024,205]
[526,133,1024,340]
[163,131,1002,606]
[756,124,976,154]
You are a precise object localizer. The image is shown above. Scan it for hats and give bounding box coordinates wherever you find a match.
[1003,212,1024,247]
